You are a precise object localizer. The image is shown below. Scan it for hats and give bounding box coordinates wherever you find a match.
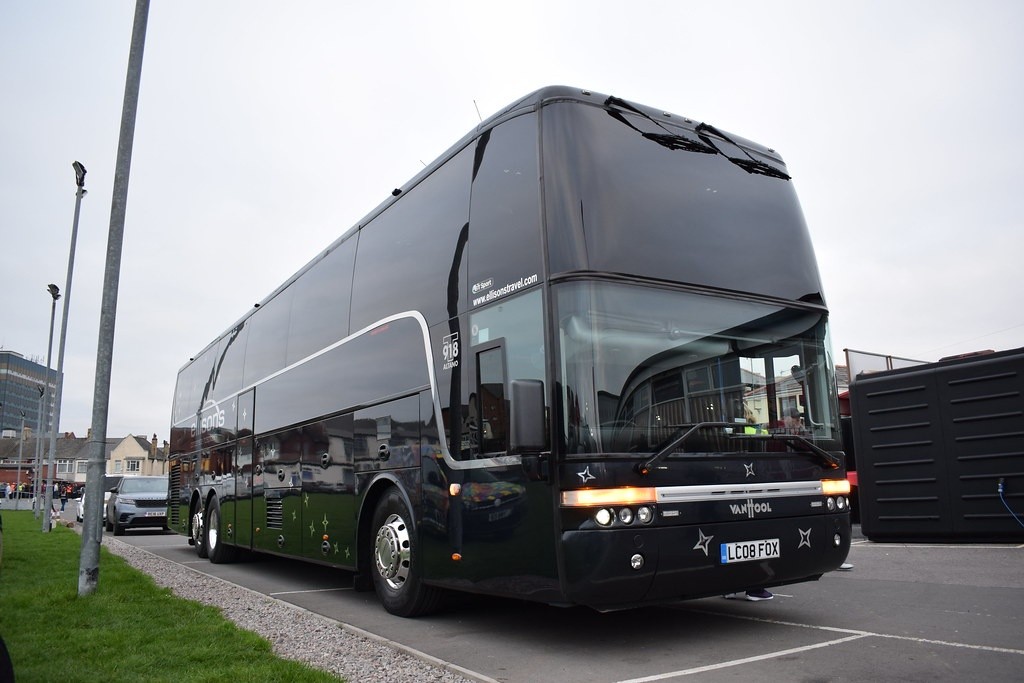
[782,408,806,417]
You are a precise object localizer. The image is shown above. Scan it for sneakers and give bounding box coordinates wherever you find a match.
[837,563,854,570]
[723,590,774,601]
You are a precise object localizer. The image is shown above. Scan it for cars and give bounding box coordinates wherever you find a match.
[104,473,137,523]
[77,493,84,522]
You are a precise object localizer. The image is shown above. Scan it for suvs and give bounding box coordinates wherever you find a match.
[104,475,172,536]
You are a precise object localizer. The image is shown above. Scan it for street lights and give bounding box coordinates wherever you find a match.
[44,162,90,535]
[32,385,44,513]
[36,284,61,520]
[16,410,26,512]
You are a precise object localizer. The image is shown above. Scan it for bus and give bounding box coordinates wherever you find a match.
[165,93,853,623]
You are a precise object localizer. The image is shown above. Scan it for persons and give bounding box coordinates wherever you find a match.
[60,487,67,511]
[743,404,769,435]
[6,480,85,500]
[782,408,806,430]
